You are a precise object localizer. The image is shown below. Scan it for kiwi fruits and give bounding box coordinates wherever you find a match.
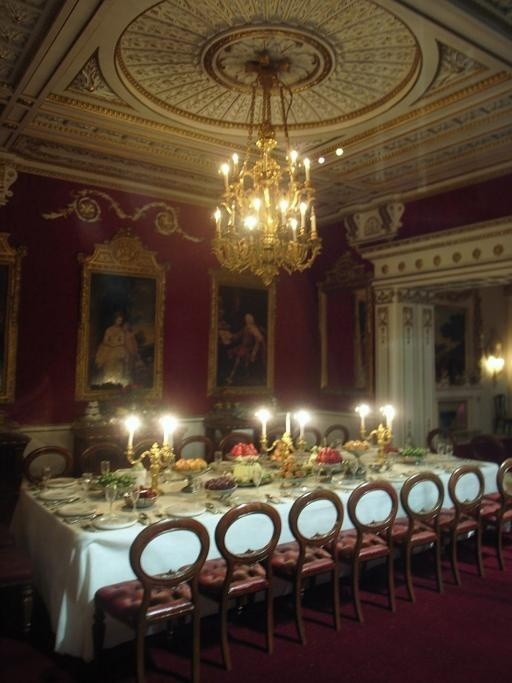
[277,454,310,480]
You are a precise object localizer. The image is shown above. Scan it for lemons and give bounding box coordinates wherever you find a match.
[98,474,139,489]
[174,456,209,473]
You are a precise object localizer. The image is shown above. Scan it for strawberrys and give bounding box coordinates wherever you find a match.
[315,447,343,465]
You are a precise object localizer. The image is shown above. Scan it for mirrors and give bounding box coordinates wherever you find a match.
[314,250,375,402]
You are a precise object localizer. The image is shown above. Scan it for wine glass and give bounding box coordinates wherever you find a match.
[40,443,455,520]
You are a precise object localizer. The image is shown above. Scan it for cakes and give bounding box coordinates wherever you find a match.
[230,460,269,484]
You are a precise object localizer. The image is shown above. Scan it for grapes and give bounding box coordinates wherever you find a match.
[203,476,236,491]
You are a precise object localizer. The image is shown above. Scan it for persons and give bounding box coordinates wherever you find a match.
[223,313,263,386]
[95,313,138,375]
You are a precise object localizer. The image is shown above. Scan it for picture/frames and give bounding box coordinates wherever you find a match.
[204,264,281,401]
[0,231,30,407]
[352,287,371,391]
[434,294,482,402]
[72,225,168,403]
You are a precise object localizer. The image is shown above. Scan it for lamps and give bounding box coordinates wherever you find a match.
[477,323,507,388]
[210,55,325,290]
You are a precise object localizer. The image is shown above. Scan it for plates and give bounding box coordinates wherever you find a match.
[37,455,459,531]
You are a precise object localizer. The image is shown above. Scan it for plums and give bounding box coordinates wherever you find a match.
[229,441,260,458]
